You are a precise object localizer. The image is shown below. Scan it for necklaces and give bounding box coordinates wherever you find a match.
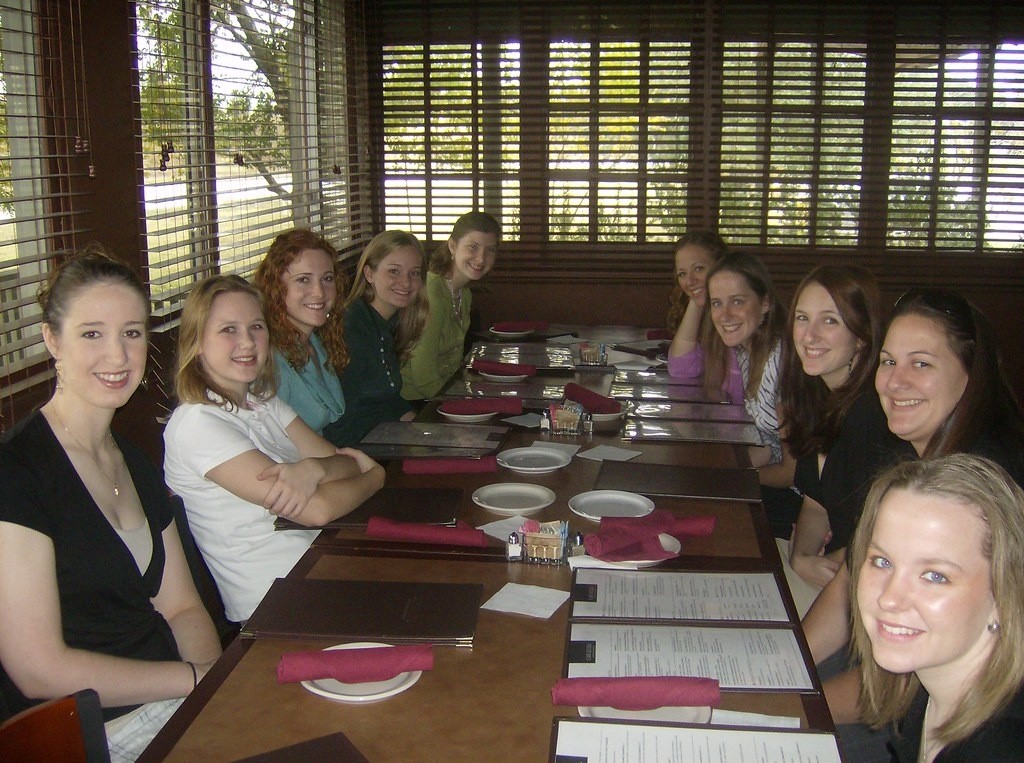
[49,400,119,497]
[447,276,463,322]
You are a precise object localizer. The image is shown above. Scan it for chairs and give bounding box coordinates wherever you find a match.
[0,688,112,763]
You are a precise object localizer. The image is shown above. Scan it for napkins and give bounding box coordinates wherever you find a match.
[277,644,433,685]
[564,383,621,414]
[601,516,717,536]
[473,358,536,376]
[403,456,497,474]
[365,515,489,548]
[551,676,721,712]
[583,514,679,562]
[647,329,669,340]
[493,321,549,331]
[442,397,522,415]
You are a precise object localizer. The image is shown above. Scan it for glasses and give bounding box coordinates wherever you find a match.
[892,287,962,319]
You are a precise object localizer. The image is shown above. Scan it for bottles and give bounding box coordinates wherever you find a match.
[540,405,593,435]
[569,344,608,366]
[508,530,585,565]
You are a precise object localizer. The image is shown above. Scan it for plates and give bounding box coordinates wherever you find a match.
[568,490,655,523]
[593,409,627,421]
[471,483,557,516]
[489,326,536,337]
[495,447,571,474]
[436,405,498,422]
[478,370,528,383]
[300,640,422,705]
[578,703,711,724]
[620,533,681,567]
[655,353,669,367]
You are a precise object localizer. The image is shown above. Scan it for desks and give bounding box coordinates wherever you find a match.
[135,322,848,763]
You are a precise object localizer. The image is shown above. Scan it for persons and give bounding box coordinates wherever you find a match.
[400,212,503,411]
[785,284,1024,725]
[765,260,913,556]
[252,229,368,447]
[341,231,430,448]
[1,242,244,763]
[706,249,797,511]
[162,274,386,628]
[667,230,744,406]
[833,452,1024,763]
